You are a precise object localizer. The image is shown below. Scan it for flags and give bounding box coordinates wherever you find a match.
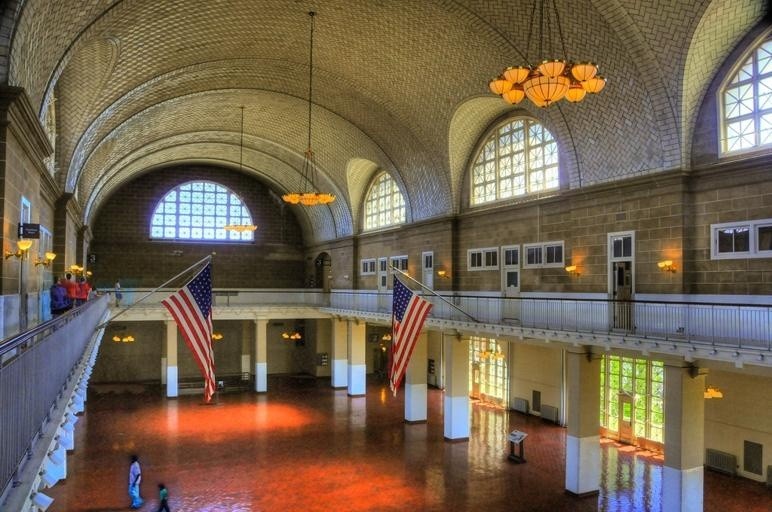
[159,259,217,405]
[387,275,434,399]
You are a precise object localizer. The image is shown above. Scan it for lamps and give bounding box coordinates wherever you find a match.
[565,265,580,275]
[700,384,723,399]
[279,11,336,206]
[488,2,608,110]
[224,105,258,233]
[657,260,676,273]
[437,270,450,279]
[6,240,93,279]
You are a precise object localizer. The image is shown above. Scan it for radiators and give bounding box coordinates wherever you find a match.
[705,448,736,474]
[513,397,558,422]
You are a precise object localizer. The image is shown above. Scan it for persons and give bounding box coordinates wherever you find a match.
[156,483,170,512]
[305,274,318,288]
[115,279,123,307]
[88,286,98,301]
[50,275,73,320]
[128,454,145,509]
[75,277,90,307]
[61,273,81,309]
